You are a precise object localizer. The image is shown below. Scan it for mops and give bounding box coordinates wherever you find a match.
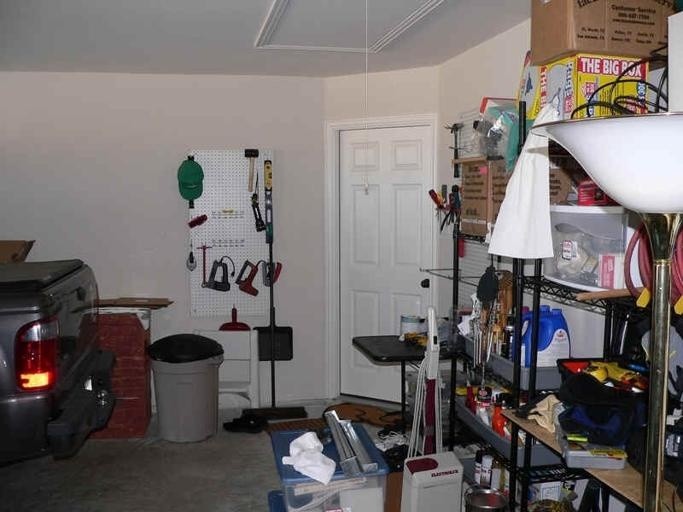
[242,228,309,419]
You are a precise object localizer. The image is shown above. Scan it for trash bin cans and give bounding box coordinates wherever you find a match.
[147,333,224,444]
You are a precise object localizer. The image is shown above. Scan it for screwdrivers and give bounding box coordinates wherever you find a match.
[429,185,460,225]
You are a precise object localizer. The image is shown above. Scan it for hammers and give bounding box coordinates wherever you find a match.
[245,148,259,192]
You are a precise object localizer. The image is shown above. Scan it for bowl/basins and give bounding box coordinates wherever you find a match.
[0,240,35,264]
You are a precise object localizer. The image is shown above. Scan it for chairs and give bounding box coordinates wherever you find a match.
[189,327,262,418]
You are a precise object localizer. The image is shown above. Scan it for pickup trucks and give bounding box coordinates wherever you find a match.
[0,258,117,464]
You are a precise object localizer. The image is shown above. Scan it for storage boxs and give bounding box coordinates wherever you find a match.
[268,420,390,512]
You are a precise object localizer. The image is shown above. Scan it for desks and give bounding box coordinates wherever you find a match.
[351,330,450,436]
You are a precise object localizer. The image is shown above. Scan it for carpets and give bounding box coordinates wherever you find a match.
[265,399,427,474]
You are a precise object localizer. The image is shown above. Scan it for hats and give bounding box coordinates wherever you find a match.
[176,159,204,202]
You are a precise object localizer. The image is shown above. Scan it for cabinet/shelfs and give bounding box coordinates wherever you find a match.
[434,111,682,512]
[82,303,154,442]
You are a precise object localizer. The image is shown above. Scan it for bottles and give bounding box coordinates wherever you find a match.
[474,447,509,496]
[502,316,514,360]
[466,384,514,438]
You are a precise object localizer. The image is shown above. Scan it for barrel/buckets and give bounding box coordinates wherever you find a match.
[531,308,571,368]
[511,304,550,367]
[464,481,508,512]
